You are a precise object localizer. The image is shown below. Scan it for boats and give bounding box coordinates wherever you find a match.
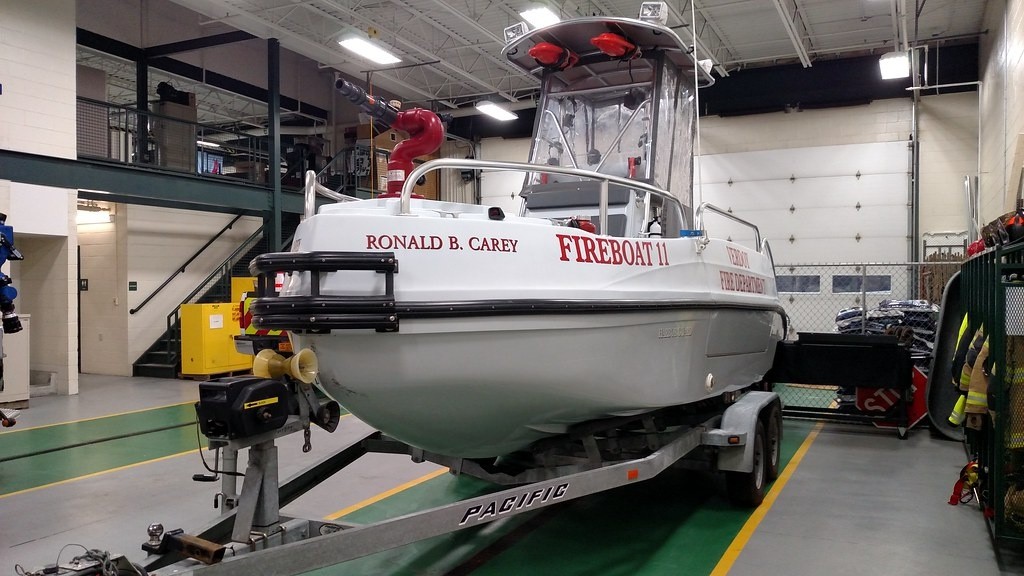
[279,0,788,462]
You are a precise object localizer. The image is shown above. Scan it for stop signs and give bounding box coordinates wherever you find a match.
[854,363,928,430]
